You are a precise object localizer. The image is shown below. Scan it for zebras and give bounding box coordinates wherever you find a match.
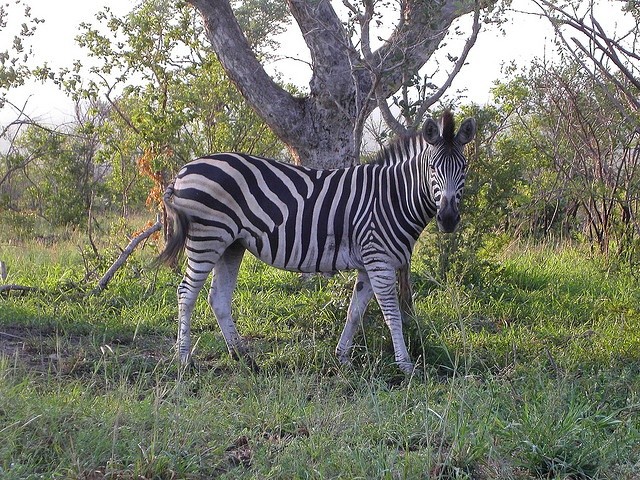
[143,112,477,376]
